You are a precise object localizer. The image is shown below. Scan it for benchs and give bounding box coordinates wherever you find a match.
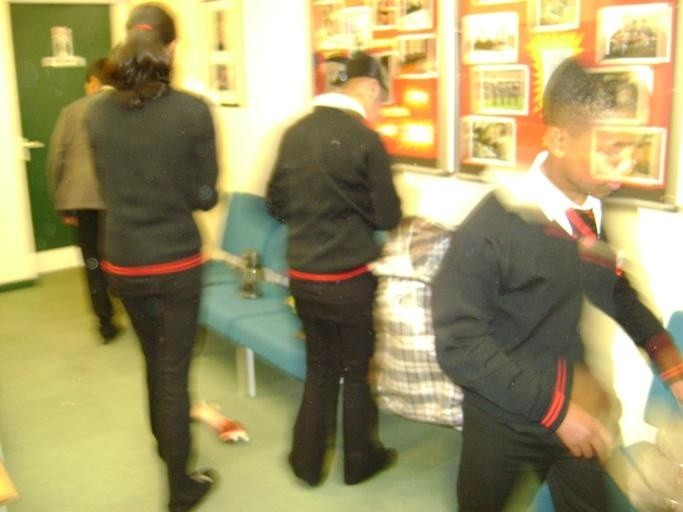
[197,191,388,396]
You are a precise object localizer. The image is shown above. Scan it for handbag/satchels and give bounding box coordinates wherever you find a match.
[368,215,464,426]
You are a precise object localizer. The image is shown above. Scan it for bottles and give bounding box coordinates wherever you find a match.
[238,247,265,299]
[50,25,76,62]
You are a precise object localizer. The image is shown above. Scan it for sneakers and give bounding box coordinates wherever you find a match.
[99,322,117,338]
[169,471,212,510]
[289,452,323,485]
[347,449,397,483]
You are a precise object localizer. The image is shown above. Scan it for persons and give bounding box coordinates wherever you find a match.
[466,15,660,180]
[79,1,219,511]
[263,50,401,486]
[43,57,125,343]
[428,54,683,511]
